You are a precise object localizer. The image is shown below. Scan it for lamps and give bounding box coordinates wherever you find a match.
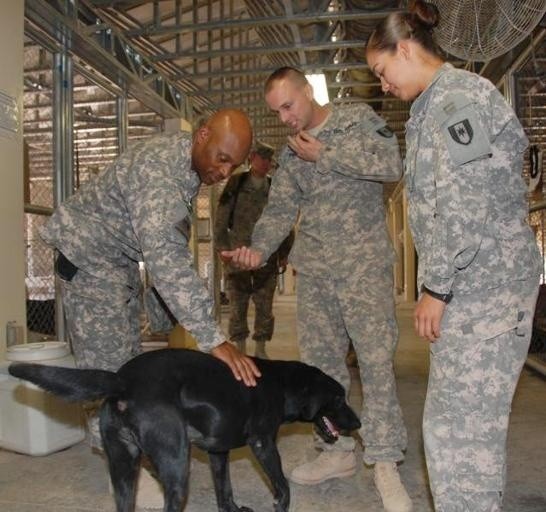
[304,70,329,106]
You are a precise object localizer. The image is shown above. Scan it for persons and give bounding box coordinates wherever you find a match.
[367,1,543,511]
[39,106,261,461]
[219,140,295,363]
[221,68,413,512]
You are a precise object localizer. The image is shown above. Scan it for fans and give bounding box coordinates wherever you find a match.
[424,0,546,63]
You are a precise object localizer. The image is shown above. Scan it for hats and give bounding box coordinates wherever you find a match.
[251,139,276,161]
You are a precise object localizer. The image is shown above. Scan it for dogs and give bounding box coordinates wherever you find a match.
[7,347,362,512]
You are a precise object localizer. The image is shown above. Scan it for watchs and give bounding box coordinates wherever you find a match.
[422,285,454,305]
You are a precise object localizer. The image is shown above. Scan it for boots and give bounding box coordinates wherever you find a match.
[253,340,271,362]
[290,449,358,487]
[236,337,247,355]
[107,463,168,510]
[373,458,414,512]
